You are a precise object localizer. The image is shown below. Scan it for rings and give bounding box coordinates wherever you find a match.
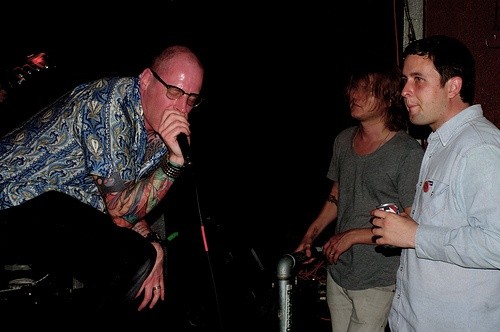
[153,285,163,290]
[330,252,335,255]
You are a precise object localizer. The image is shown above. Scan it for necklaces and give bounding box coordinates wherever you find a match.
[373,130,391,154]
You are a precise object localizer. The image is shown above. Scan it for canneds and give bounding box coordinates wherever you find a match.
[376,203,401,249]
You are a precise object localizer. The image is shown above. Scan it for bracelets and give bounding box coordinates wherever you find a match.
[161,158,183,181]
[145,232,161,243]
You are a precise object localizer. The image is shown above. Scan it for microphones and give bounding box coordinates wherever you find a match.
[177,132,192,164]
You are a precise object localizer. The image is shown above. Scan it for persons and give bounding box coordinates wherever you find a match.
[0,45,204,321]
[369,33,500,332]
[293,60,425,332]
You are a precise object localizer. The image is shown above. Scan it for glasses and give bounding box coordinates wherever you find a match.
[150,69,204,107]
